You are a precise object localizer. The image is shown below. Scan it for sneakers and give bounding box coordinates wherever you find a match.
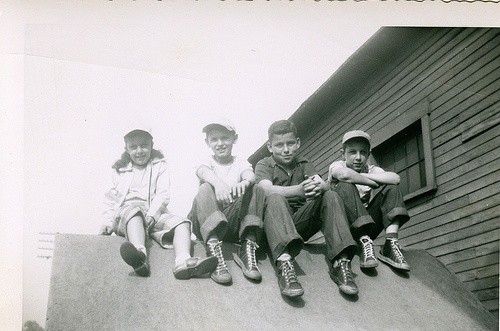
[231,238,262,281]
[359,234,378,268]
[377,238,410,271]
[276,257,305,297]
[329,253,359,295]
[206,239,232,283]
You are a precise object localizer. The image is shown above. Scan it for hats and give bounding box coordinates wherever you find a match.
[202,119,236,134]
[343,130,372,152]
[123,121,156,145]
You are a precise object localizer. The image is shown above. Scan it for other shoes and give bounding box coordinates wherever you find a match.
[119,241,149,276]
[172,255,218,279]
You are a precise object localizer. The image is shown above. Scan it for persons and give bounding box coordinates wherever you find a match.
[326,129,411,270]
[188,119,267,286]
[254,120,360,297]
[97,123,218,279]
